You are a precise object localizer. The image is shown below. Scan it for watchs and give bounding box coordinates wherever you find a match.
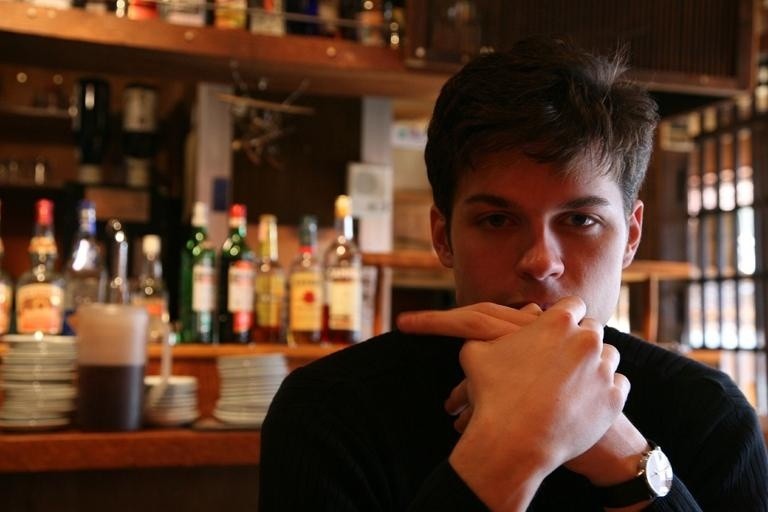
[592,439,678,508]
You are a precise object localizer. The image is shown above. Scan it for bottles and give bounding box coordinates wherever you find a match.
[1,244,13,336]
[30,1,407,59]
[133,233,170,341]
[216,204,258,344]
[66,78,111,166]
[120,83,161,190]
[96,219,132,303]
[178,201,219,342]
[251,214,288,342]
[62,206,107,338]
[284,215,325,341]
[15,198,64,335]
[322,193,363,341]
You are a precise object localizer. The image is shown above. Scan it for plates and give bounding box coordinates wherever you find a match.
[143,374,199,428]
[213,353,289,428]
[1,338,77,431]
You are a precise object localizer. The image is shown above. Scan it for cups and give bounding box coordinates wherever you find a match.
[81,303,146,432]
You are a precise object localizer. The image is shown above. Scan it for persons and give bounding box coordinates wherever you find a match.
[259,39,766,510]
